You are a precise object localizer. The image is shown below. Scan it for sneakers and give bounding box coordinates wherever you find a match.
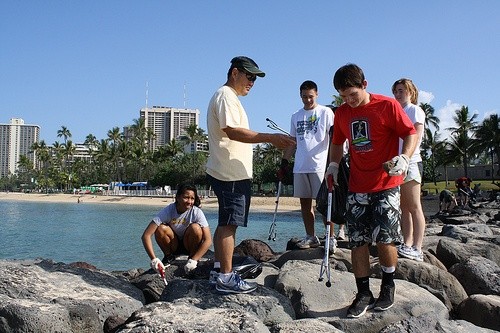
[300,235,321,248]
[374,281,395,310]
[162,254,175,267]
[337,229,345,239]
[346,290,375,318]
[323,234,336,241]
[324,237,337,254]
[396,243,423,262]
[209,267,258,293]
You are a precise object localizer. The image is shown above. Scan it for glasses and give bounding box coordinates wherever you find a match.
[239,70,257,82]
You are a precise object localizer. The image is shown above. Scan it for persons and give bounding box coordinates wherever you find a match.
[278,80,340,252]
[439,189,459,212]
[324,64,419,318]
[390,78,426,262]
[454,176,472,206]
[207,55,296,293]
[142,183,212,273]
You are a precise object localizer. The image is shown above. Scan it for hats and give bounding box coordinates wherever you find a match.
[230,55,265,78]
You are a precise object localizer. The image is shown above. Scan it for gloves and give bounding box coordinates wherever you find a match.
[150,258,165,274]
[184,258,197,273]
[325,162,340,190]
[389,155,410,181]
